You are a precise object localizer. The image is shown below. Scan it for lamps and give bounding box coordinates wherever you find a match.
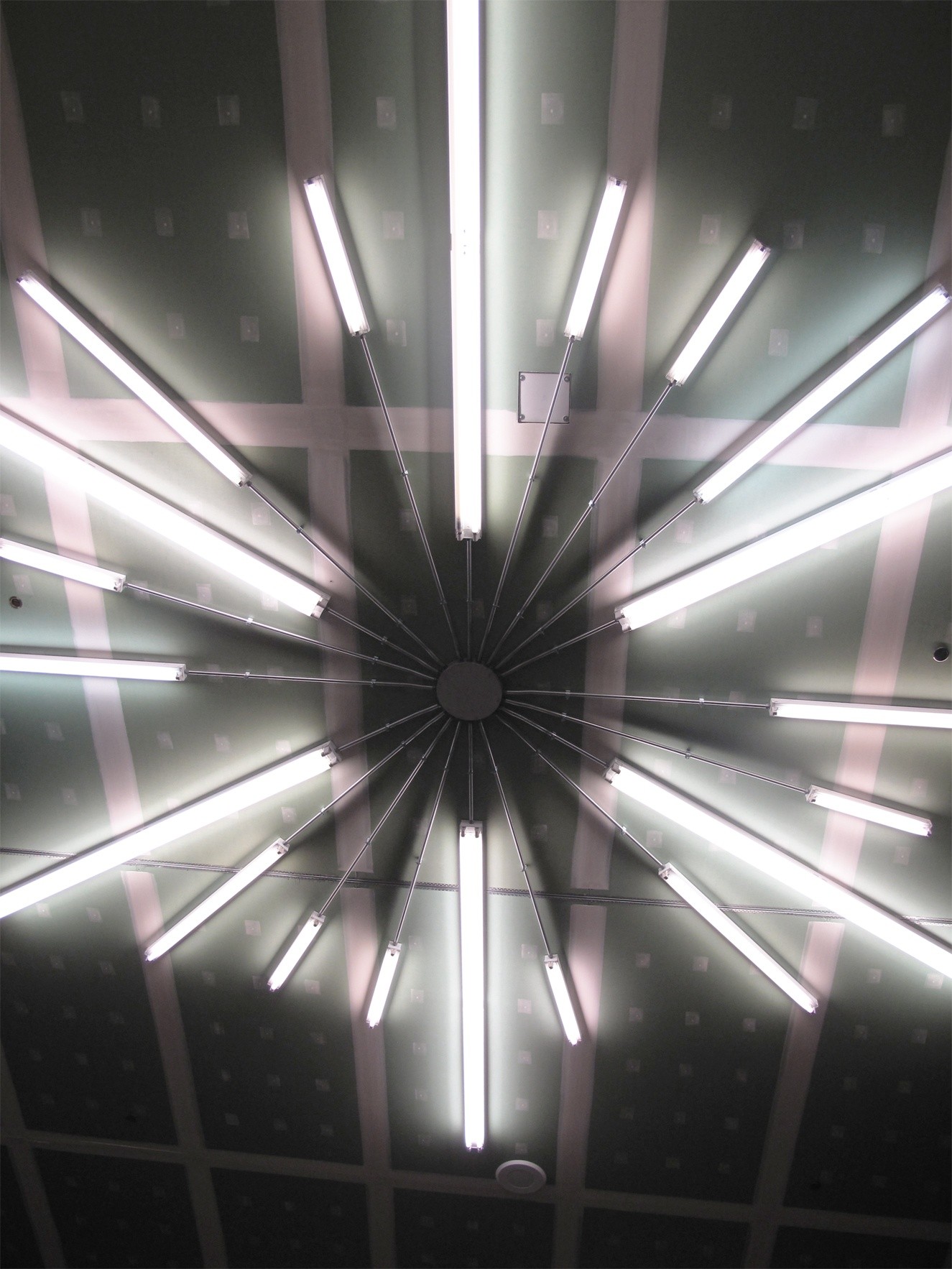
[0,0,952,1155]
